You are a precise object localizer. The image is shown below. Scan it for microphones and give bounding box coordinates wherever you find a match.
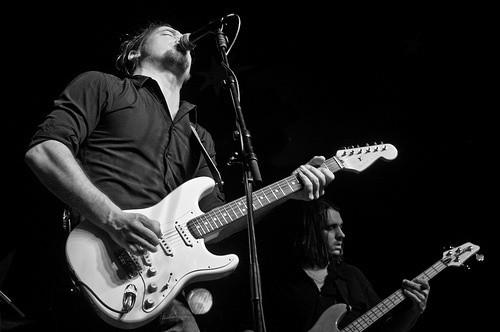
[179,14,236,50]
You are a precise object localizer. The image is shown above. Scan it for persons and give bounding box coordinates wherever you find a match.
[24,22,335,331]
[264,201,431,332]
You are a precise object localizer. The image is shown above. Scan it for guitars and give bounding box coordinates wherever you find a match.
[305,240,485,331]
[65,140,400,328]
